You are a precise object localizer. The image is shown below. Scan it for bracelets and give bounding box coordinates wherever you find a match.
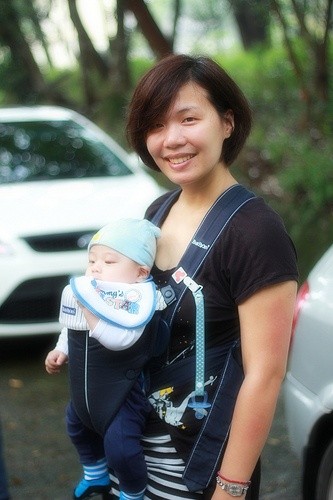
[217,471,251,484]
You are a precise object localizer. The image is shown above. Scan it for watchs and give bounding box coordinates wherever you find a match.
[216,477,249,496]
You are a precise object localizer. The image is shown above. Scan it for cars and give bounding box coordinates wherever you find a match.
[256,246,333,500]
[0,105,170,336]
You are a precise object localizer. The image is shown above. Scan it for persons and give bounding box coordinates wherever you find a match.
[44,219,171,500]
[125,55,299,500]
